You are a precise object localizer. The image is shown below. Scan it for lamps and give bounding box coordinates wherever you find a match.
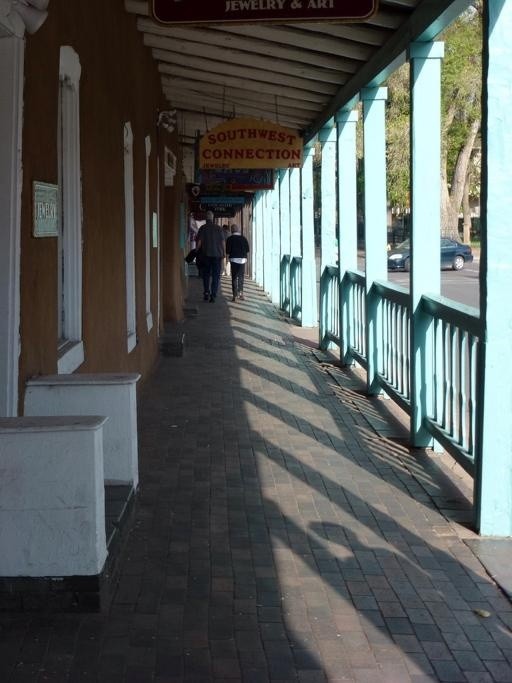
[157,108,177,132]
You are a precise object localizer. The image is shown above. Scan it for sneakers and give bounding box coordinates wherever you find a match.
[232,291,245,302]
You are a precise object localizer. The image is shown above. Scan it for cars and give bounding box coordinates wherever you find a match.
[387,236,473,272]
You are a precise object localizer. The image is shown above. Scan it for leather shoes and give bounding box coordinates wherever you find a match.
[203,293,216,303]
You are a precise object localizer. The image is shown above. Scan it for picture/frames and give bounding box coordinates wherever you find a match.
[32,180,63,238]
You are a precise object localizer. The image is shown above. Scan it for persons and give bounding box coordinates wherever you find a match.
[187,206,250,302]
